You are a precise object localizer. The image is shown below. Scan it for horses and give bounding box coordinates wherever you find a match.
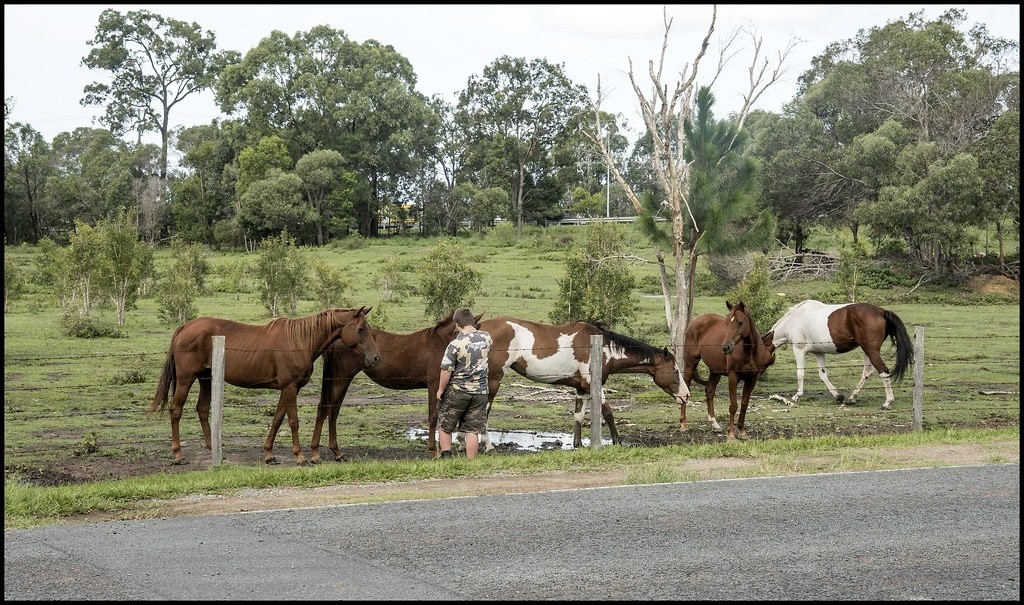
[762,299,916,409]
[679,300,765,441]
[310,308,487,465]
[479,316,690,448]
[145,305,381,467]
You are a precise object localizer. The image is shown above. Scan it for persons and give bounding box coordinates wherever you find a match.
[435,306,494,462]
[381,217,388,231]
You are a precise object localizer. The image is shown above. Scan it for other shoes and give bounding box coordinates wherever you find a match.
[439,450,452,459]
